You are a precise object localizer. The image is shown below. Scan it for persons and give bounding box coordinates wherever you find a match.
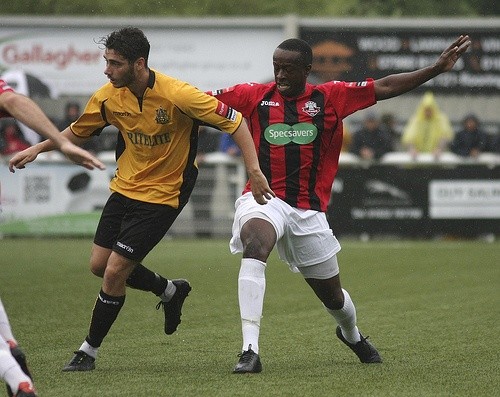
[8,27,277,373]
[340,93,500,166]
[54,99,102,153]
[201,38,458,372]
[0,336,37,396]
[0,78,108,396]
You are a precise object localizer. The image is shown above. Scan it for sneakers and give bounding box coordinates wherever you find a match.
[5,338,32,396]
[62,349,95,371]
[336,323,382,364]
[156,278,191,334]
[232,348,262,373]
